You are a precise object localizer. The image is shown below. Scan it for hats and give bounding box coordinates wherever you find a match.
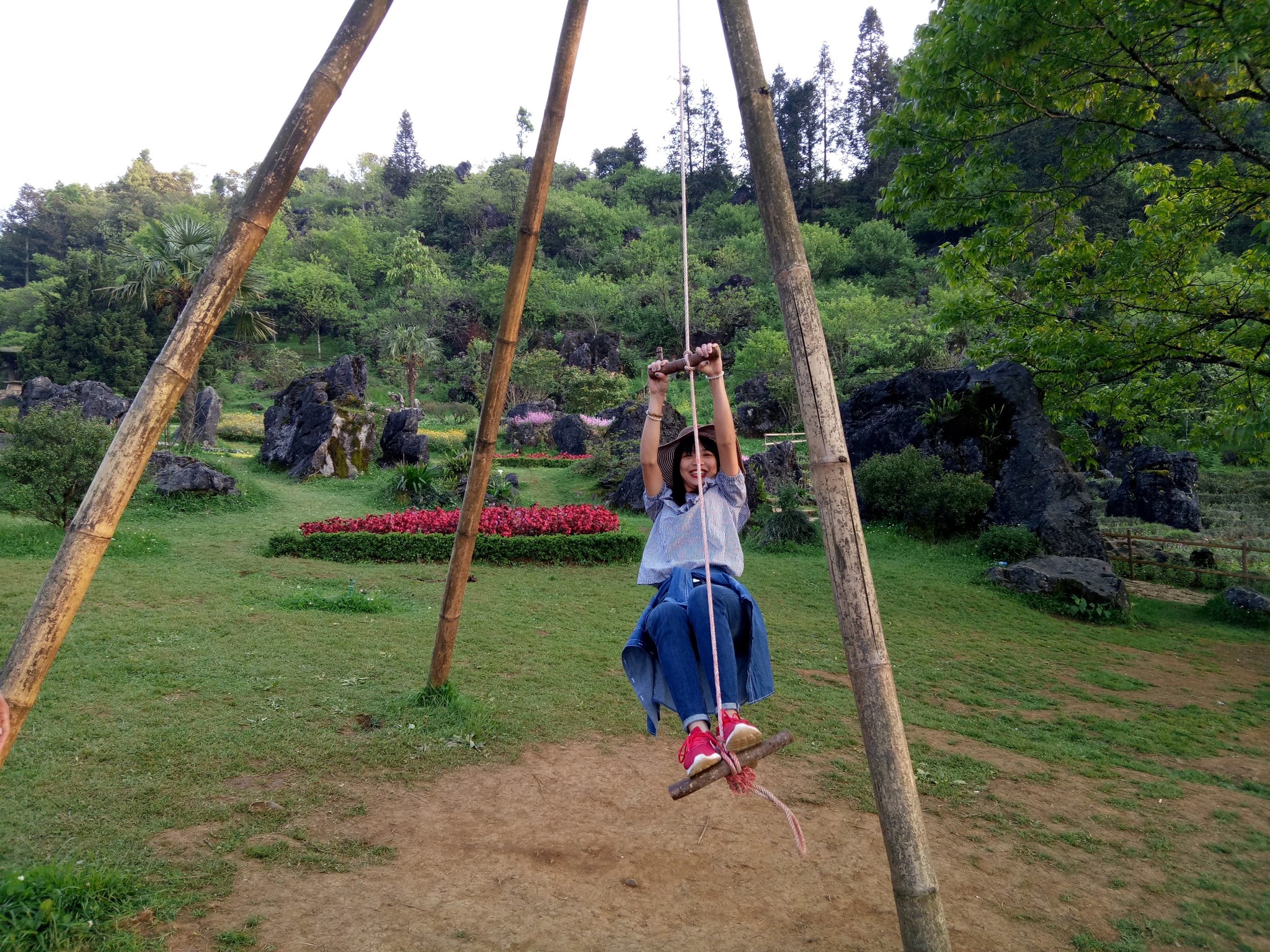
[657,424,745,489]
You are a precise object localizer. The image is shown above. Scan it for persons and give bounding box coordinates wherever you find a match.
[620,343,777,777]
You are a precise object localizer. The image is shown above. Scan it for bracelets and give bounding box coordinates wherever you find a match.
[645,410,663,421]
[705,371,724,382]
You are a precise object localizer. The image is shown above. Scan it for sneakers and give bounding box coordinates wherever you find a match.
[678,727,722,777]
[715,708,763,769]
